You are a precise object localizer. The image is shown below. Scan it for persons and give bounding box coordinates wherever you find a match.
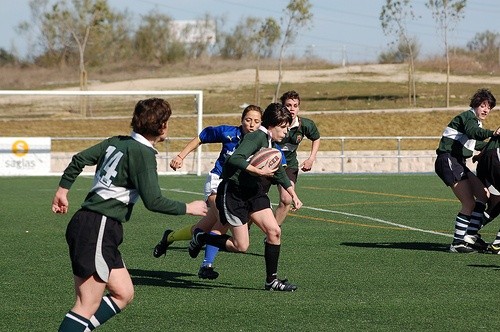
[248,90,320,246]
[52,98,208,332]
[189,103,304,291]
[153,104,287,280]
[435,88,500,254]
[465,134,500,255]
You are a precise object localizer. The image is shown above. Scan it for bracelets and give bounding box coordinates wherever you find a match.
[178,155,183,160]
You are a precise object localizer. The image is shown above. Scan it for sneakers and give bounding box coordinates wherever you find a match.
[189,228,208,258]
[265,278,296,291]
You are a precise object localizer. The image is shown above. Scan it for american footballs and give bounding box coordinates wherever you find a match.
[248,148,282,176]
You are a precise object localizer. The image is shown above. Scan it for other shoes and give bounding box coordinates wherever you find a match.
[450,241,477,254]
[476,233,488,246]
[465,239,486,251]
[153,229,174,258]
[484,245,500,254]
[198,266,219,279]
